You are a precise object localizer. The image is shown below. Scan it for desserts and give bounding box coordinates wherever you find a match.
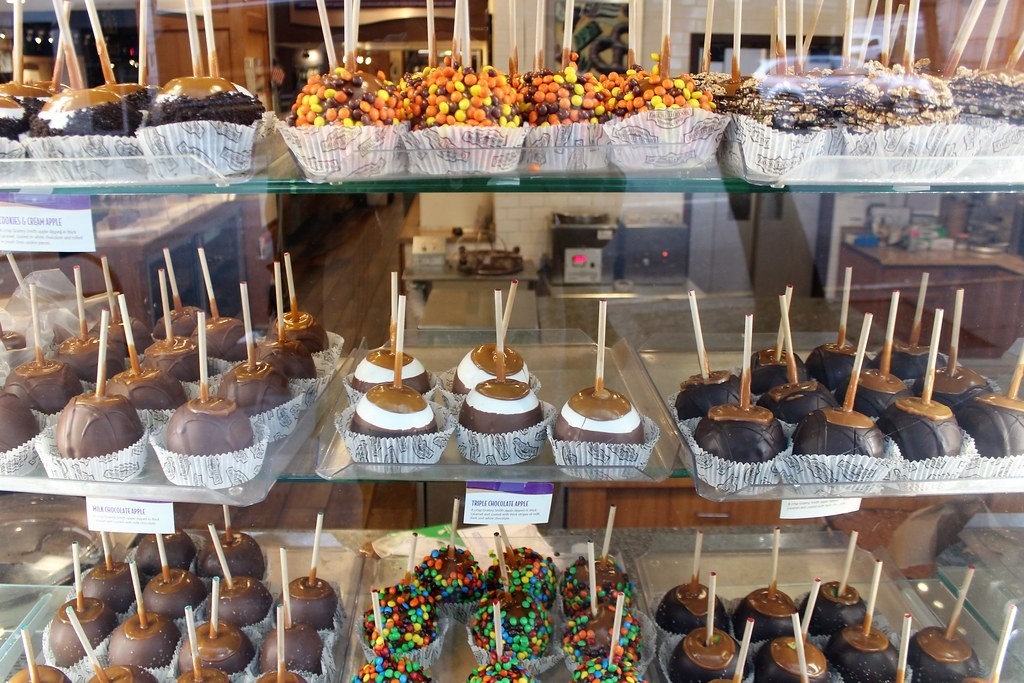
[5,533,980,683]
[0,304,1024,490]
[0,64,1024,185]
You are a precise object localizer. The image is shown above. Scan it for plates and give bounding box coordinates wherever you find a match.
[971,245,1007,254]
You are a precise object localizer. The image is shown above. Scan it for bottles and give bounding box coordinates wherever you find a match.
[954,232,969,251]
[906,230,919,252]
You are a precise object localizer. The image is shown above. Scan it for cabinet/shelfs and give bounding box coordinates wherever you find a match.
[0,107,1024,683]
